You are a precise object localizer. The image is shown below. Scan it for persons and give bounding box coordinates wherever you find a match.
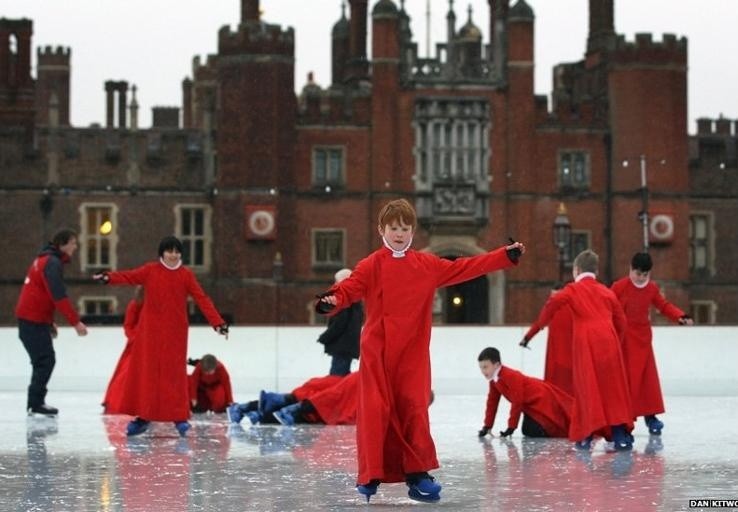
[521,250,637,450]
[102,284,144,407]
[477,346,576,438]
[316,268,363,376]
[315,200,525,497]
[16,230,88,414]
[224,371,434,425]
[610,252,694,430]
[92,234,230,437]
[186,355,233,413]
[546,281,573,392]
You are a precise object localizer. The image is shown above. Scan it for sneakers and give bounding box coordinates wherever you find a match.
[358,481,378,497]
[612,430,632,449]
[126,417,149,433]
[174,421,193,435]
[27,403,58,415]
[407,473,442,497]
[648,418,663,434]
[228,390,305,425]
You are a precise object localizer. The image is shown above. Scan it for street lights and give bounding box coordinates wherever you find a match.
[38,186,52,251]
[550,201,573,292]
[273,250,284,326]
